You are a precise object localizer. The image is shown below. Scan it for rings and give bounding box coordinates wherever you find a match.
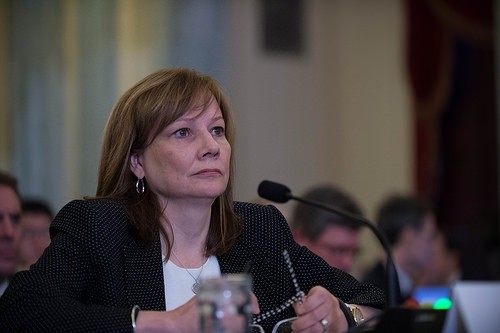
[321,319,329,333]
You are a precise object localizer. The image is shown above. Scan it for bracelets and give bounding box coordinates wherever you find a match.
[131,305,141,333]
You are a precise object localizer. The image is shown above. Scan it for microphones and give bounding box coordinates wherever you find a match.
[257,180,449,333]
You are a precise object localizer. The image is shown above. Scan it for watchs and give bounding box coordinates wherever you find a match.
[345,303,365,327]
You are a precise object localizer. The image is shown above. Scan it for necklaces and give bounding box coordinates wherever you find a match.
[160,234,204,295]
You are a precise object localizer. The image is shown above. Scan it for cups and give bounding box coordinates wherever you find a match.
[198,274,251,333]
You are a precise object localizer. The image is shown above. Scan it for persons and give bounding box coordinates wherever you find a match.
[0,68,392,333]
[359,193,460,309]
[0,173,54,297]
[290,186,364,271]
